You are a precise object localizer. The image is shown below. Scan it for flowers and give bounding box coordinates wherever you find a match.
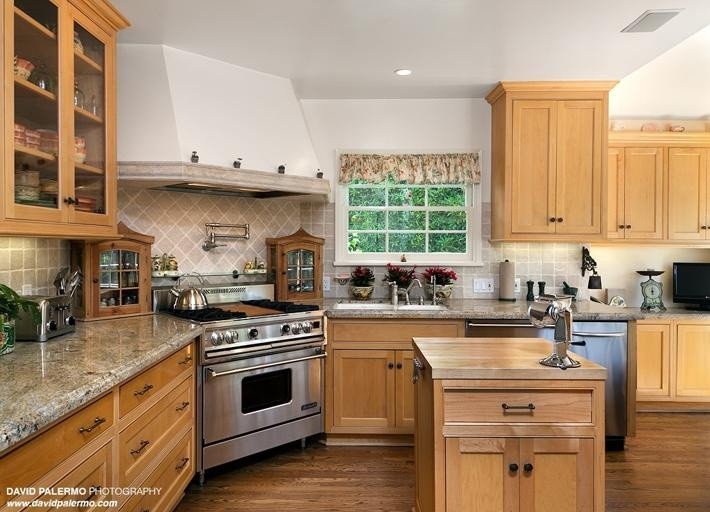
[382,263,418,290]
[420,267,457,286]
[349,265,375,287]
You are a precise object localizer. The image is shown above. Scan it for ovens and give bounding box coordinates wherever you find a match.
[196,310,327,488]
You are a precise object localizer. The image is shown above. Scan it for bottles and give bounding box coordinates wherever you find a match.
[100,295,136,306]
[91,94,99,115]
[152,254,178,271]
[74,80,81,107]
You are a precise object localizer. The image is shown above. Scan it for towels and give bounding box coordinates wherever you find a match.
[498,261,516,301]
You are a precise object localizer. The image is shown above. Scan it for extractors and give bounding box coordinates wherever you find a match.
[119,44,330,199]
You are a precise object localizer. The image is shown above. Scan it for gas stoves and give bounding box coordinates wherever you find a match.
[158,300,319,323]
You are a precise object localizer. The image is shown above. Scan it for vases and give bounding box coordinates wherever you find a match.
[424,283,452,300]
[350,285,374,301]
[389,284,410,301]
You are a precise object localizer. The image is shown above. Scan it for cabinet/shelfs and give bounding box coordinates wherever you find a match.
[0,336,196,512]
[321,318,466,448]
[483,79,710,251]
[637,319,710,414]
[70,222,155,323]
[0,0,133,241]
[266,226,325,302]
[412,347,605,512]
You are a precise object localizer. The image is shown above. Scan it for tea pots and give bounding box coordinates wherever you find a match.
[168,271,207,311]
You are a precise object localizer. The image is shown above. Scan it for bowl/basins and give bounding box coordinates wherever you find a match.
[14,58,36,81]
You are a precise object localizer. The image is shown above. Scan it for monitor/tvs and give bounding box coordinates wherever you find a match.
[673,262,710,311]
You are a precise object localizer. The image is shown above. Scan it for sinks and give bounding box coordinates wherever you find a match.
[331,298,449,312]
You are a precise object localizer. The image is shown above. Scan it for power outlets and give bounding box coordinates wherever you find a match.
[485,278,494,294]
[323,277,331,291]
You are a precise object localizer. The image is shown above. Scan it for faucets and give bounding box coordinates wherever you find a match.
[387,277,423,305]
[429,274,438,305]
[203,223,250,243]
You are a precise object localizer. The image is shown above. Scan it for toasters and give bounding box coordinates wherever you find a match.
[13,294,76,343]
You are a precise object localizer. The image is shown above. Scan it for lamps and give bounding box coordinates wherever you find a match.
[581,246,602,289]
